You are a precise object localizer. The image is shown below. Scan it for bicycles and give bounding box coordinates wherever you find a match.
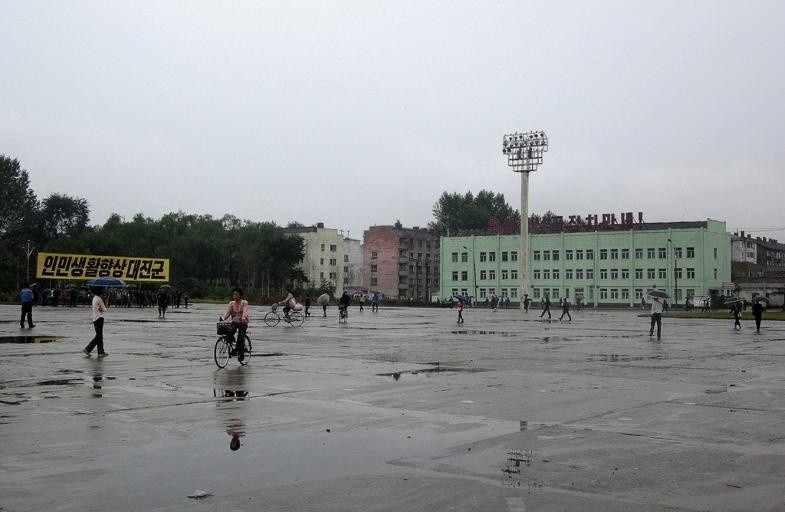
[215,320,252,369]
[338,304,348,323]
[265,303,305,329]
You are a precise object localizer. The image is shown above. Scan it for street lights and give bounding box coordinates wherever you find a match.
[462,245,477,307]
[665,236,678,305]
[22,239,36,284]
[503,129,548,306]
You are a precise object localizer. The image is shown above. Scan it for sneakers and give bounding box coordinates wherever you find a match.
[83,349,91,356]
[238,352,244,361]
[99,353,108,357]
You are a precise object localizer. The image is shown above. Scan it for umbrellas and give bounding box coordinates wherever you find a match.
[86,276,127,288]
[317,293,330,306]
[647,289,669,298]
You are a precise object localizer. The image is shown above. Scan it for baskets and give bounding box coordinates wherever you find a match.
[217,322,233,335]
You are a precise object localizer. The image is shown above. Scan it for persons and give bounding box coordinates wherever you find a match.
[303,293,311,317]
[83,286,108,359]
[278,289,296,323]
[339,291,351,318]
[220,288,249,362]
[662,299,668,312]
[323,303,329,317]
[642,295,668,340]
[436,292,571,324]
[19,282,35,329]
[33,283,189,318]
[372,293,380,312]
[358,294,365,312]
[642,297,645,305]
[685,298,763,332]
